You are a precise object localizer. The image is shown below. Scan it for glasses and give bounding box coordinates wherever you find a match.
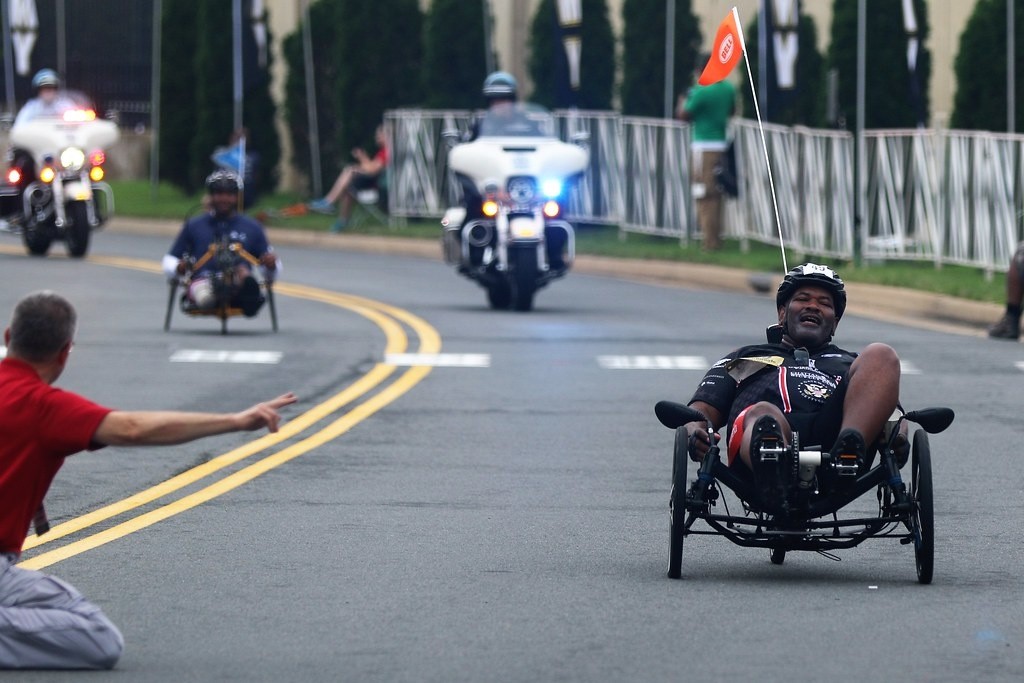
[59,339,75,355]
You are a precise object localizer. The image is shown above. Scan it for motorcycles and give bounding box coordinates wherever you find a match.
[439,101,598,314]
[3,92,123,260]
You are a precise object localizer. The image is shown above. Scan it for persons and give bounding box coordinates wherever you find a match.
[809,359,815,368]
[0,292,299,669]
[677,51,737,255]
[990,239,1024,340]
[11,68,84,225]
[680,262,914,501]
[162,171,283,317]
[310,125,391,235]
[457,73,542,261]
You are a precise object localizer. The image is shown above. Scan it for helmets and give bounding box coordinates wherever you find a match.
[481,70,518,96]
[32,68,61,88]
[205,168,244,194]
[777,262,847,324]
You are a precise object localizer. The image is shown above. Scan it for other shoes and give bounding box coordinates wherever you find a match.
[239,276,261,317]
[189,279,214,309]
[989,316,1021,339]
[750,415,788,514]
[822,429,866,505]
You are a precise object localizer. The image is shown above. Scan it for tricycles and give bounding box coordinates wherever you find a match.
[666,420,935,585]
[163,203,280,337]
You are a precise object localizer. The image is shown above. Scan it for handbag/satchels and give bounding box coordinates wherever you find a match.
[713,142,738,198]
